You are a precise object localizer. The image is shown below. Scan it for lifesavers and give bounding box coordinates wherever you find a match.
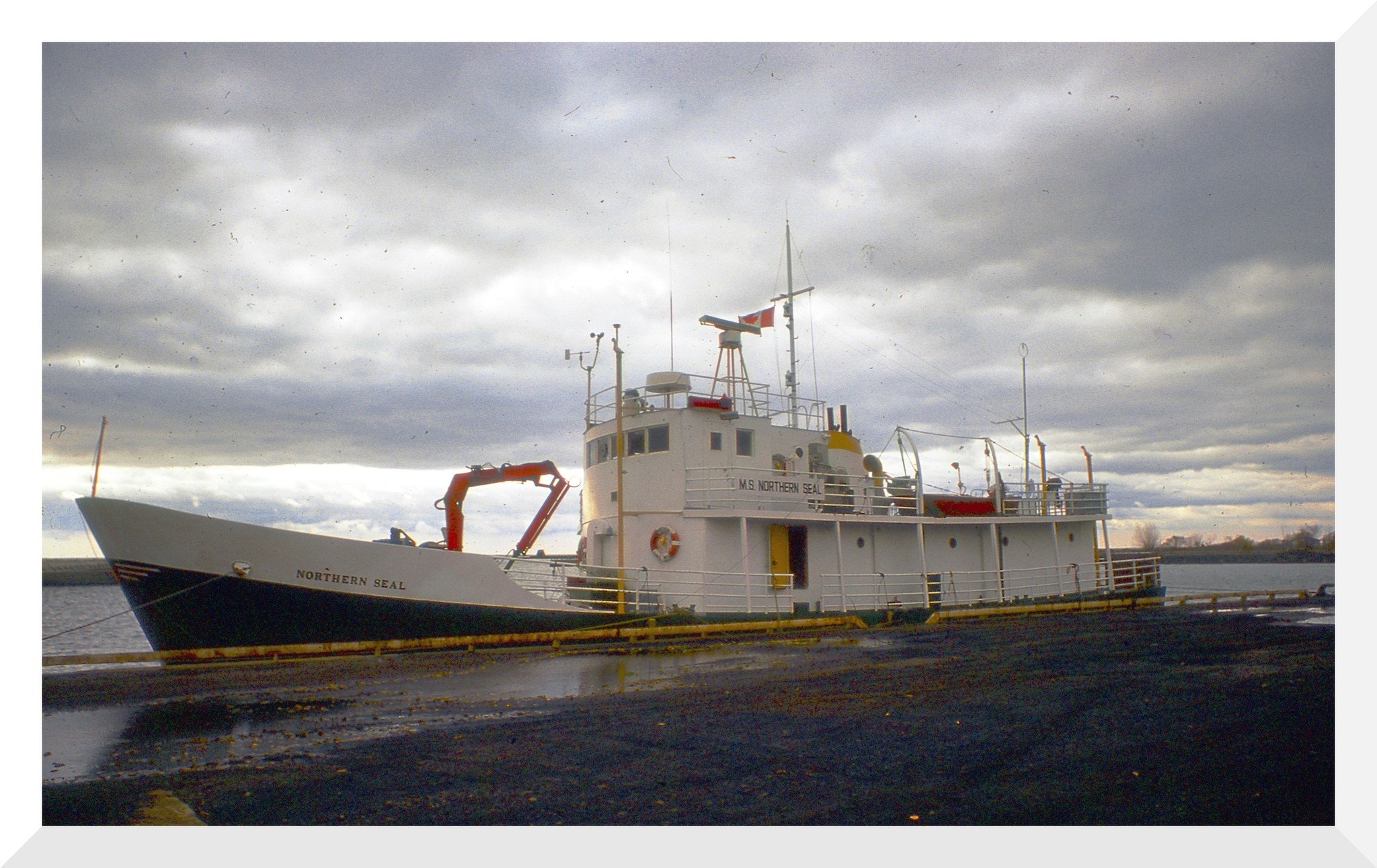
[649,525,679,561]
[577,535,587,568]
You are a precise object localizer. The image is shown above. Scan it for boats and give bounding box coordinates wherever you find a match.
[69,215,1168,717]
